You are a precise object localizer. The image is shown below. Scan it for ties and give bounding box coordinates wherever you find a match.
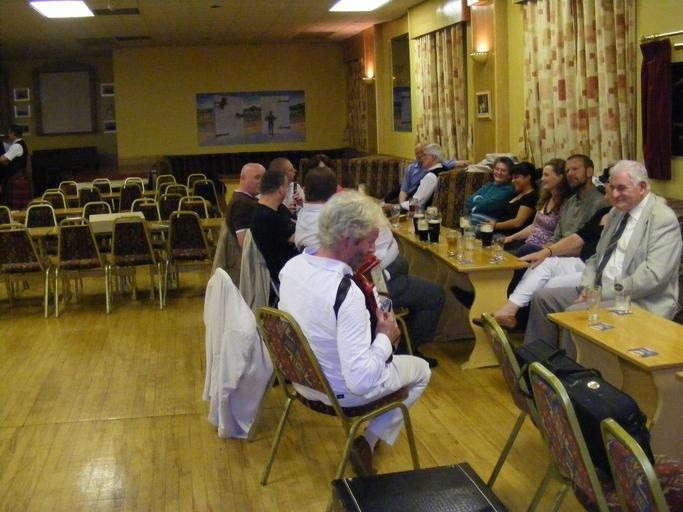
[594,212,630,291]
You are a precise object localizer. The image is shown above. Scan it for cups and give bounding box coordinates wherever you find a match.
[390,198,442,244]
[613,275,634,315]
[584,284,602,323]
[445,215,505,265]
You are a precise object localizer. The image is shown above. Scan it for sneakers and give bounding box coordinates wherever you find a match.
[412,350,438,368]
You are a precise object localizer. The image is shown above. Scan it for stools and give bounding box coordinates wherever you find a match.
[331,461,508,512]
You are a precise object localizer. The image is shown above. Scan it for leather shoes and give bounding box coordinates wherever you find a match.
[348,435,376,476]
[472,312,518,331]
[450,284,474,309]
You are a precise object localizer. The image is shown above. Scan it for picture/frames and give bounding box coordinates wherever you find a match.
[100,82,114,97]
[14,89,30,101]
[104,119,117,133]
[15,105,31,119]
[474,91,491,121]
[15,122,31,136]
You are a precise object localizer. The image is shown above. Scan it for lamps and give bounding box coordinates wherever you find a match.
[470,52,489,66]
[363,78,374,86]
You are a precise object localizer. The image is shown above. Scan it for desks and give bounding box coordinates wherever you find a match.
[390,221,529,371]
[546,308,683,467]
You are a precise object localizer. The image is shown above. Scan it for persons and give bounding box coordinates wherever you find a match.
[226,153,442,480]
[382,144,448,213]
[264,110,276,136]
[463,154,682,362]
[0,125,28,177]
[399,142,471,204]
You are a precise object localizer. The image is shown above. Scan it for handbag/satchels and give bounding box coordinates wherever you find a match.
[514,338,654,477]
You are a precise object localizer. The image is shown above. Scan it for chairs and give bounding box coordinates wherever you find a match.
[600,417,674,512]
[394,306,414,356]
[528,362,681,512]
[0,173,229,316]
[256,307,419,512]
[201,268,291,443]
[482,313,554,512]
